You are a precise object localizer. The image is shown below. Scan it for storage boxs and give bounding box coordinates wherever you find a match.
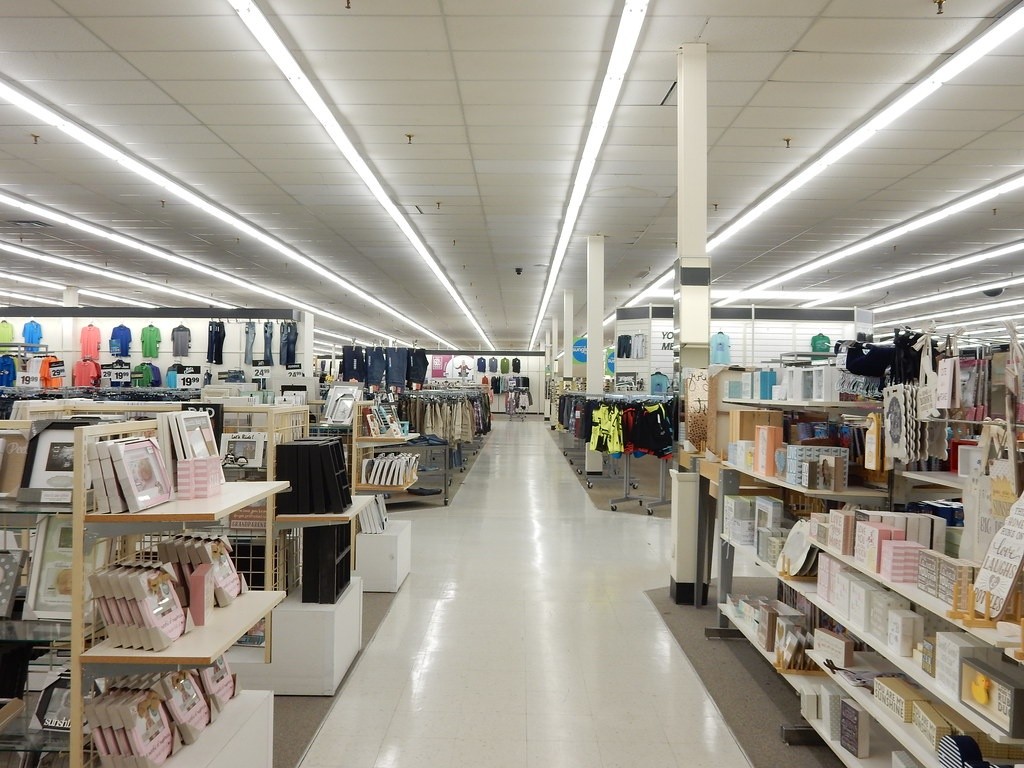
[0,405,419,768]
[723,426,1024,768]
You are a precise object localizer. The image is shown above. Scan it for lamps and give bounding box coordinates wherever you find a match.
[62,287,80,308]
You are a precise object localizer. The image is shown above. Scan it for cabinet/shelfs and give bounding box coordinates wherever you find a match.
[191,399,420,593]
[366,401,450,508]
[705,396,888,638]
[773,471,1024,768]
[0,397,291,768]
[15,404,375,697]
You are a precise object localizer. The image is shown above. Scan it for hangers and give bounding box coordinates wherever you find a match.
[1,321,184,366]
[480,357,518,360]
[558,393,673,407]
[818,328,823,336]
[718,329,724,335]
[402,394,469,400]
